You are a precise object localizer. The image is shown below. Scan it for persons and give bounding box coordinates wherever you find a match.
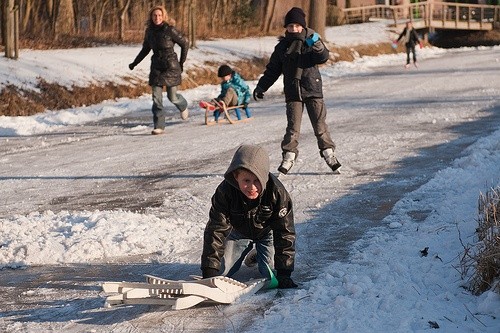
[253,5,342,178]
[200,64,253,116]
[128,5,191,133]
[200,142,300,289]
[395,20,422,69]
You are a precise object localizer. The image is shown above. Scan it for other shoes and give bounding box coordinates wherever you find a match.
[218,101,226,112]
[152,128,165,135]
[199,100,215,111]
[180,107,188,120]
[244,248,259,267]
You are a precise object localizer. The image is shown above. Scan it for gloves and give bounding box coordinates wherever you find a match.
[178,61,183,72]
[277,270,297,288]
[129,61,137,70]
[311,40,323,52]
[253,85,265,101]
[240,102,249,109]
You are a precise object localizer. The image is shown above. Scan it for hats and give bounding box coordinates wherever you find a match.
[217,65,233,77]
[283,6,307,29]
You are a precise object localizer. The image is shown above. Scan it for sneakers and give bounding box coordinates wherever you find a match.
[320,147,342,174]
[276,151,296,179]
